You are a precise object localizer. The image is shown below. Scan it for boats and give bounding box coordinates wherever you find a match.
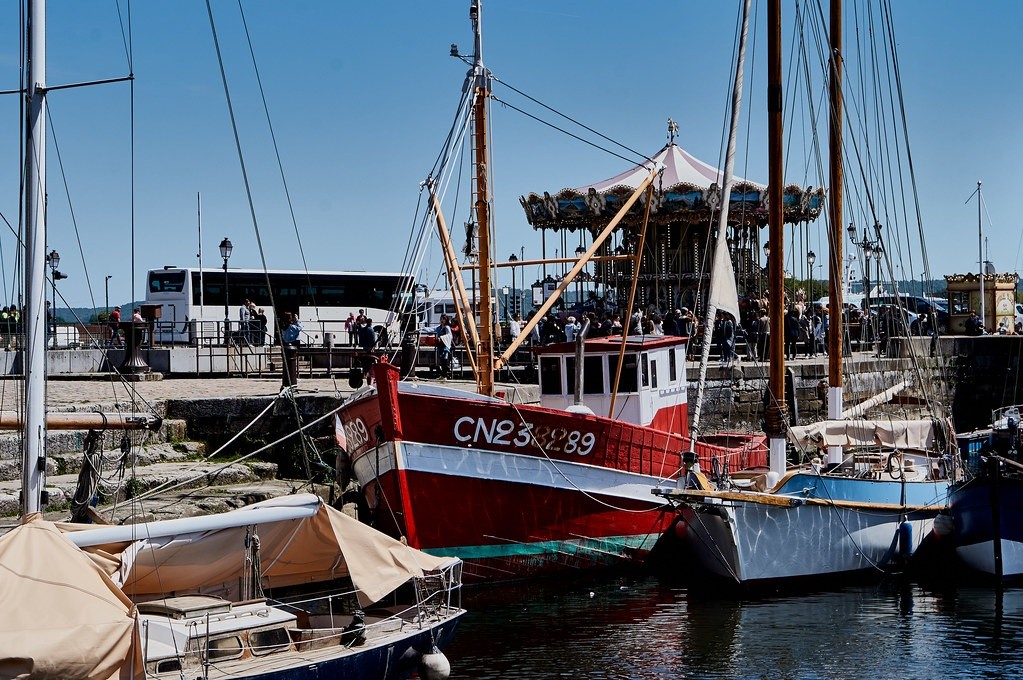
[935,403,1023,582]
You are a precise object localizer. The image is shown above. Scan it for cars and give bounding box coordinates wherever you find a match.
[68,341,127,350]
[1014,303,1023,332]
[863,304,927,334]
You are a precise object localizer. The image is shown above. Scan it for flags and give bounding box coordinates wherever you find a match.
[707,236,743,328]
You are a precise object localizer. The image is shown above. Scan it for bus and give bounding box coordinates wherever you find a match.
[145,267,430,346]
[402,290,480,328]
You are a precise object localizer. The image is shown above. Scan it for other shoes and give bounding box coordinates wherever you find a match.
[809,354,817,359]
[806,353,810,360]
[442,377,447,383]
[436,376,442,380]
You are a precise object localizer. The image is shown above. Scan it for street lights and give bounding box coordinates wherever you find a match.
[218,238,233,346]
[574,246,586,306]
[106,275,112,344]
[48,250,61,350]
[507,254,518,316]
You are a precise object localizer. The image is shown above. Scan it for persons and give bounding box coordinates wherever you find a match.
[527,309,698,362]
[716,288,947,363]
[131,308,145,350]
[108,306,125,349]
[510,312,521,366]
[964,312,983,336]
[359,318,376,376]
[0,300,52,352]
[240,298,267,347]
[435,314,460,380]
[281,312,303,365]
[345,308,368,346]
[996,322,1007,335]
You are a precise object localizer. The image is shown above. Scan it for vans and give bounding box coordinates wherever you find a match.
[927,298,961,314]
[48,326,80,348]
[862,297,949,334]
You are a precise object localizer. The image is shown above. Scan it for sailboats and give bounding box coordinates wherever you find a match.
[0,0,468,680]
[685,1,980,584]
[326,1,766,585]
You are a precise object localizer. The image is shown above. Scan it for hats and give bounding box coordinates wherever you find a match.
[115,306,121,309]
